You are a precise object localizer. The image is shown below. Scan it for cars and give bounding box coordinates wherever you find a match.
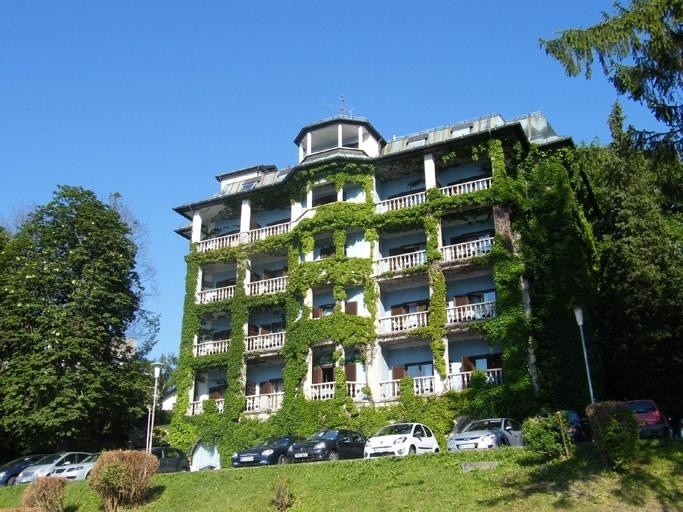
[363,421,440,459]
[231,435,306,468]
[285,428,367,463]
[623,399,671,438]
[49,452,103,482]
[540,409,585,445]
[14,451,92,485]
[0,452,48,487]
[136,446,190,473]
[446,417,526,451]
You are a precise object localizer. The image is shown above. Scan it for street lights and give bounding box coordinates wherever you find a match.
[573,305,597,404]
[144,360,165,455]
